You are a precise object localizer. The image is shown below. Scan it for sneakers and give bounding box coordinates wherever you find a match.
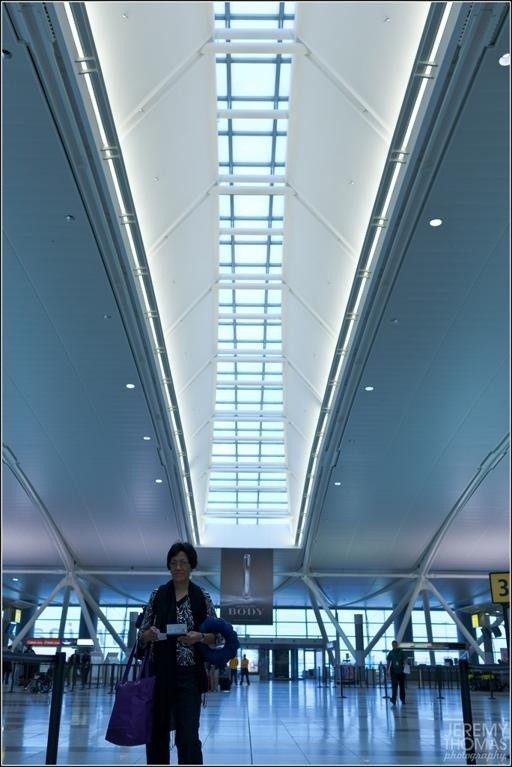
[231,682,251,685]
[390,698,406,706]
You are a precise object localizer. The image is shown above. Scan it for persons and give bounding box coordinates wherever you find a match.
[69,649,80,683]
[229,657,238,686]
[2,646,12,684]
[138,542,238,764]
[239,654,250,686]
[81,651,91,685]
[387,641,408,705]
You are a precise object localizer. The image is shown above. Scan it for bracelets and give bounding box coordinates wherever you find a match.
[200,632,205,643]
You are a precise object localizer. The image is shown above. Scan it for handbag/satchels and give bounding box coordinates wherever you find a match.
[104,638,156,747]
[403,663,411,675]
